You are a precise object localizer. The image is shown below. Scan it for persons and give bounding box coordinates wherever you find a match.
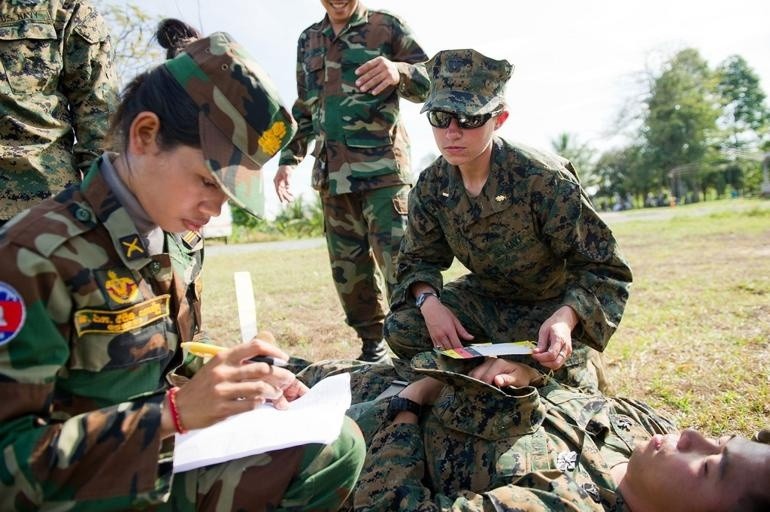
[1,1,123,234]
[271,1,434,358]
[0,19,367,512]
[605,190,672,210]
[381,51,634,389]
[262,356,769,510]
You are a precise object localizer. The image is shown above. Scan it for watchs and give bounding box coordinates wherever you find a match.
[384,394,423,425]
[414,289,445,310]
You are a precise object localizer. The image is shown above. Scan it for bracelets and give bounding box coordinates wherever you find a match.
[167,386,187,434]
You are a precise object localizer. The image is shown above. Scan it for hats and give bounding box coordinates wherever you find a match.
[419,49,515,116]
[164,31,299,221]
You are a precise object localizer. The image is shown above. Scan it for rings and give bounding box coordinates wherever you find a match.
[559,352,568,359]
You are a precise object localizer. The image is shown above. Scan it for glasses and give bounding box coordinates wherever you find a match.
[426,109,504,128]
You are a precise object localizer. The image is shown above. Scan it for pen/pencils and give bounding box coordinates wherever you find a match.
[180,342,289,367]
